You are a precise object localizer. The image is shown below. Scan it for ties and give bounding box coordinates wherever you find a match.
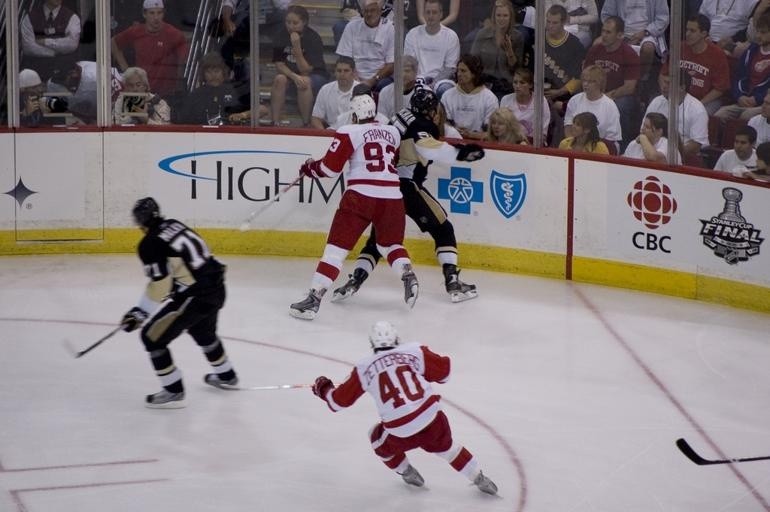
[46,10,55,28]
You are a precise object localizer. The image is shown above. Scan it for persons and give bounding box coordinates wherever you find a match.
[18,1,770,183]
[120,196,239,404]
[334,89,487,298]
[290,95,418,313]
[310,321,498,494]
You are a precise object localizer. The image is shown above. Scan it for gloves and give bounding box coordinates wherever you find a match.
[121,307,149,333]
[301,161,324,178]
[457,143,486,162]
[311,376,335,402]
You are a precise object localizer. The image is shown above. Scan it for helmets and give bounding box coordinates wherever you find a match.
[369,320,399,349]
[133,197,161,224]
[19,69,41,88]
[351,93,377,122]
[410,88,438,111]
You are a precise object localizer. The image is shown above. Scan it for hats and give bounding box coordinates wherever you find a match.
[142,0,164,9]
[220,0,234,9]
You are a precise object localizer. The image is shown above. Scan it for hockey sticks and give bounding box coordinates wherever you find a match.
[675,437,769,465]
[205,374,343,392]
[239,171,306,232]
[57,325,124,358]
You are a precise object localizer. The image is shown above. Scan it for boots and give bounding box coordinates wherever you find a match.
[445,271,478,303]
[472,469,498,495]
[332,267,368,301]
[289,288,327,320]
[401,271,419,307]
[401,465,425,488]
[145,379,188,409]
[204,368,238,385]
